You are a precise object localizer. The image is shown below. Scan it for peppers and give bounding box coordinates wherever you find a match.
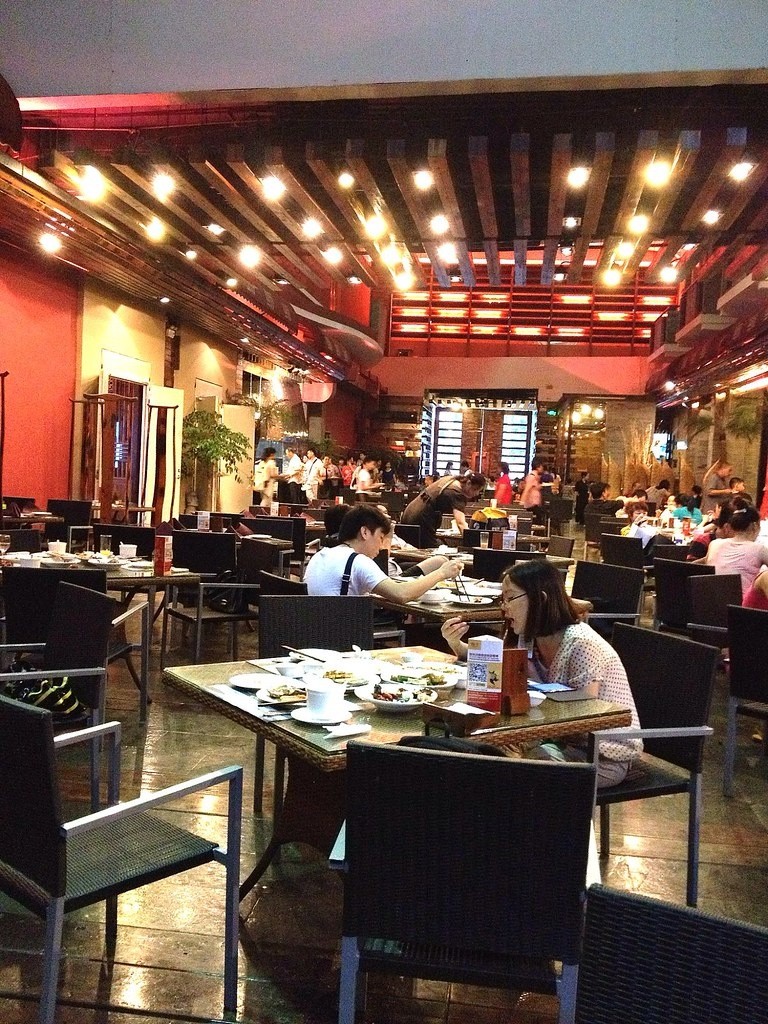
[390,673,444,685]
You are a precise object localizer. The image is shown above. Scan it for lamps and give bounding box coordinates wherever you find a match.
[650,431,676,465]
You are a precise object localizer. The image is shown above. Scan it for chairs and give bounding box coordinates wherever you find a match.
[0,490,767,1024]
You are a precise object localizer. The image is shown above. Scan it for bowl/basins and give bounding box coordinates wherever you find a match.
[354,683,438,712]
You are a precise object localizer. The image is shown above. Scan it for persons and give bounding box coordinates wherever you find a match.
[494,462,512,504]
[520,458,548,551]
[539,464,561,495]
[440,559,643,788]
[285,447,393,504]
[513,479,525,495]
[303,504,462,604]
[586,477,768,610]
[257,447,285,507]
[401,472,486,548]
[461,461,474,476]
[574,472,589,524]
[705,461,733,510]
[444,462,453,475]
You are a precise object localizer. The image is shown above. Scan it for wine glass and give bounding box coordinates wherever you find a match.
[0,534,10,555]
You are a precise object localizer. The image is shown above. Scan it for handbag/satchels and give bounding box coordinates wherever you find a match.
[5,659,87,721]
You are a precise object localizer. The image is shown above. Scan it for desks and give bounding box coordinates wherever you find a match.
[372,573,594,646]
[173,530,293,577]
[390,544,575,589]
[164,645,631,905]
[0,551,199,656]
[1,512,65,544]
[434,533,550,551]
[301,521,326,550]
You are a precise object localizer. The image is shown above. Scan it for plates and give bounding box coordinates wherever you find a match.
[391,574,503,604]
[256,684,308,706]
[276,648,467,690]
[0,551,154,571]
[290,707,353,725]
[229,673,306,689]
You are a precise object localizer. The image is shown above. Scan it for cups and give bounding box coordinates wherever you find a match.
[480,532,489,549]
[48,541,67,553]
[306,681,347,720]
[490,498,498,509]
[20,556,41,568]
[119,544,137,558]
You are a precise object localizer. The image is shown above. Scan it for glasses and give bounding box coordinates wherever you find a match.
[492,590,531,606]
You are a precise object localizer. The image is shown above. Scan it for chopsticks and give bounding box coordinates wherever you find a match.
[453,573,469,602]
[281,644,326,662]
[467,620,505,624]
